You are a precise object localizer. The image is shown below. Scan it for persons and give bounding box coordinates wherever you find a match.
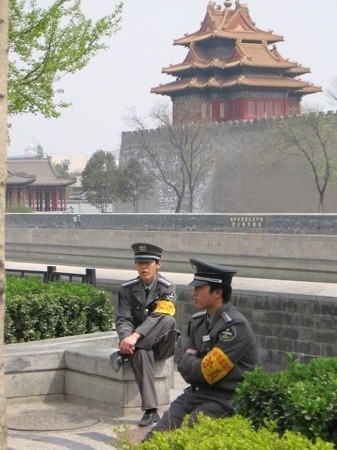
[142,256,258,445]
[109,243,181,427]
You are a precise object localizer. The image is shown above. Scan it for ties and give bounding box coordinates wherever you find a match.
[205,320,210,331]
[144,288,150,301]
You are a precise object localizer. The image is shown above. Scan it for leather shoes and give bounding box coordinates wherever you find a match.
[110,351,126,373]
[137,409,161,427]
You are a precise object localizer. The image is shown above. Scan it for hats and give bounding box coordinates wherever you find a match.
[131,242,163,262]
[187,257,238,286]
